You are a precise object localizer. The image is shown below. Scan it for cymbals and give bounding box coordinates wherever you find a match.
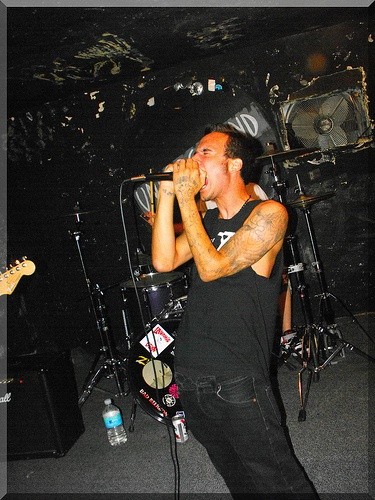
[56,208,106,217]
[285,192,335,210]
[255,148,321,164]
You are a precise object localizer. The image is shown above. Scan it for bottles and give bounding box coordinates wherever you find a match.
[101,398,127,447]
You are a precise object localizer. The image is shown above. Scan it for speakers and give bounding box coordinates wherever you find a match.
[8,347,86,461]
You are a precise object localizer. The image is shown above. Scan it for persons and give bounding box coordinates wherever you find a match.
[151,124,318,500]
[143,182,309,356]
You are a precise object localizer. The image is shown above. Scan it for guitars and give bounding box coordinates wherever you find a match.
[0,256,36,297]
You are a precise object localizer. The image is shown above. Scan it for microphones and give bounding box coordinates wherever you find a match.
[130,172,174,182]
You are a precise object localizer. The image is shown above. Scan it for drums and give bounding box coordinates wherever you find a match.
[121,272,192,431]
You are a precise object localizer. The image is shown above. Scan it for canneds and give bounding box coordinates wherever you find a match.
[171,416,189,444]
